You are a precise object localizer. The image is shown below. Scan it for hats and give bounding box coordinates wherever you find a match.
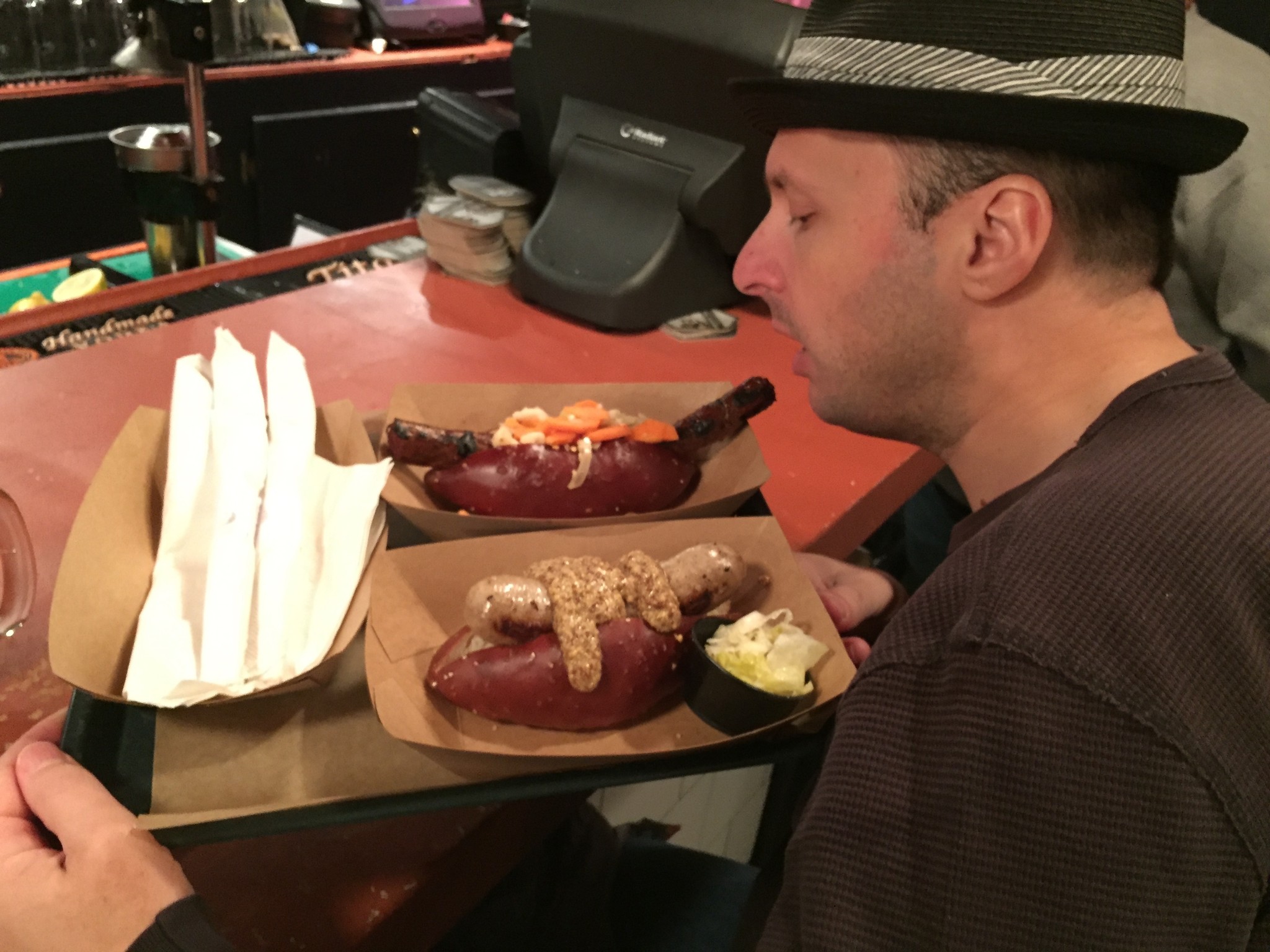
[725,0,1250,178]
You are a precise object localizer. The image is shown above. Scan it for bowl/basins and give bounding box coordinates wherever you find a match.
[677,614,813,737]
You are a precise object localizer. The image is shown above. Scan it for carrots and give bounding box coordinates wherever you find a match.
[493,399,680,450]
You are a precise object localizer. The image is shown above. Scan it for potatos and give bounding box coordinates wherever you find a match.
[424,615,720,732]
[425,443,698,518]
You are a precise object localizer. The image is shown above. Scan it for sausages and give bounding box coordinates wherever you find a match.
[386,376,777,468]
[462,541,750,638]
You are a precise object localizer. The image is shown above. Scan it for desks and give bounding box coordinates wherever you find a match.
[0,38,515,251]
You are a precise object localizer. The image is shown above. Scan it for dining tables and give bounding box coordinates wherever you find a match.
[0,256,947,951]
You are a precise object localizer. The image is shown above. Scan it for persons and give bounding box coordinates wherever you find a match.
[1,0,1270,952]
